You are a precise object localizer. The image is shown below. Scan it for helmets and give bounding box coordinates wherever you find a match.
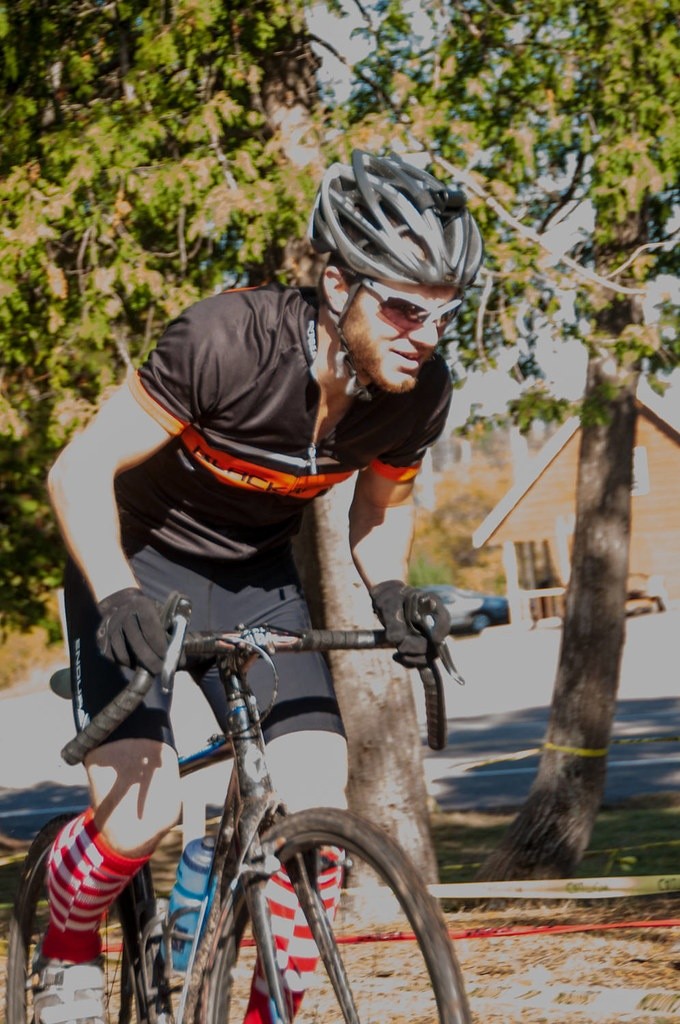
[307,147,483,290]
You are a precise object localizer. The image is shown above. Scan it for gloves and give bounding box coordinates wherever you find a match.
[370,581,451,667]
[94,588,187,675]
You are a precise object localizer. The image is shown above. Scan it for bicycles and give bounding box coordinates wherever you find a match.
[2,588,470,1023]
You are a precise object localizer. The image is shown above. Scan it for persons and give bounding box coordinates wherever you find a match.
[31,149,483,1024]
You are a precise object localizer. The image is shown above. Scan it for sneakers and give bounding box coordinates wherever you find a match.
[31,934,107,1024]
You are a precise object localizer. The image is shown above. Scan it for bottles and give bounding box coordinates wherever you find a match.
[161,836,216,969]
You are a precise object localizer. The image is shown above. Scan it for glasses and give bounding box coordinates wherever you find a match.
[338,264,464,334]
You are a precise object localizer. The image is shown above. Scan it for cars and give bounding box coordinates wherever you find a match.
[423,583,509,638]
